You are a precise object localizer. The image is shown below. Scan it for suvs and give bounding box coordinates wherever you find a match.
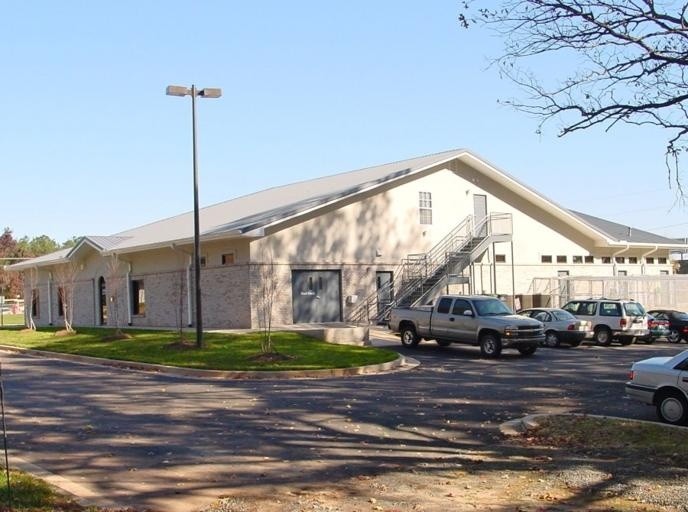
[560,298,650,345]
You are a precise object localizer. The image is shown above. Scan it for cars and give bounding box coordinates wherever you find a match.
[517,307,594,348]
[624,350,688,423]
[603,308,688,343]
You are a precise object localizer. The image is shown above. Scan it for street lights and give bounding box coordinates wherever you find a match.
[166,83,223,348]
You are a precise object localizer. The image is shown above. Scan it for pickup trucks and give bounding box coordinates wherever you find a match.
[389,295,547,357]
